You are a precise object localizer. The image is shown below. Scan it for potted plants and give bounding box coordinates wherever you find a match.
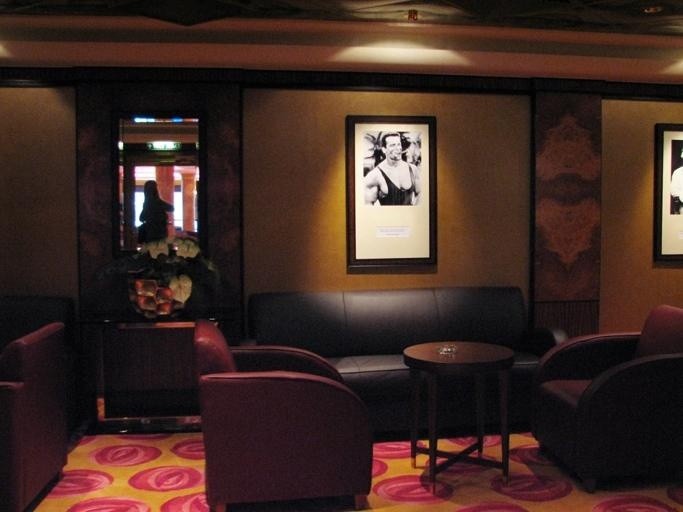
[111,236,216,321]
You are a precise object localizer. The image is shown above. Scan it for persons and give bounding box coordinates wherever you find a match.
[135,180,174,244]
[362,129,421,206]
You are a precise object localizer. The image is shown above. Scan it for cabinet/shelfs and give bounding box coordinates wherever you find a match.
[99,320,219,415]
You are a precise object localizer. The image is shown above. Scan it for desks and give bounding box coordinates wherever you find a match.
[399,340,517,497]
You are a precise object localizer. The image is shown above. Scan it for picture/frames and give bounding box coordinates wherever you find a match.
[342,113,438,270]
[651,122,683,264]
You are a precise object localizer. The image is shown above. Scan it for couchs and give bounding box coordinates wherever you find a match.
[190,317,373,512]
[526,300,683,494]
[238,285,569,446]
[0,319,71,511]
[1,293,77,360]
